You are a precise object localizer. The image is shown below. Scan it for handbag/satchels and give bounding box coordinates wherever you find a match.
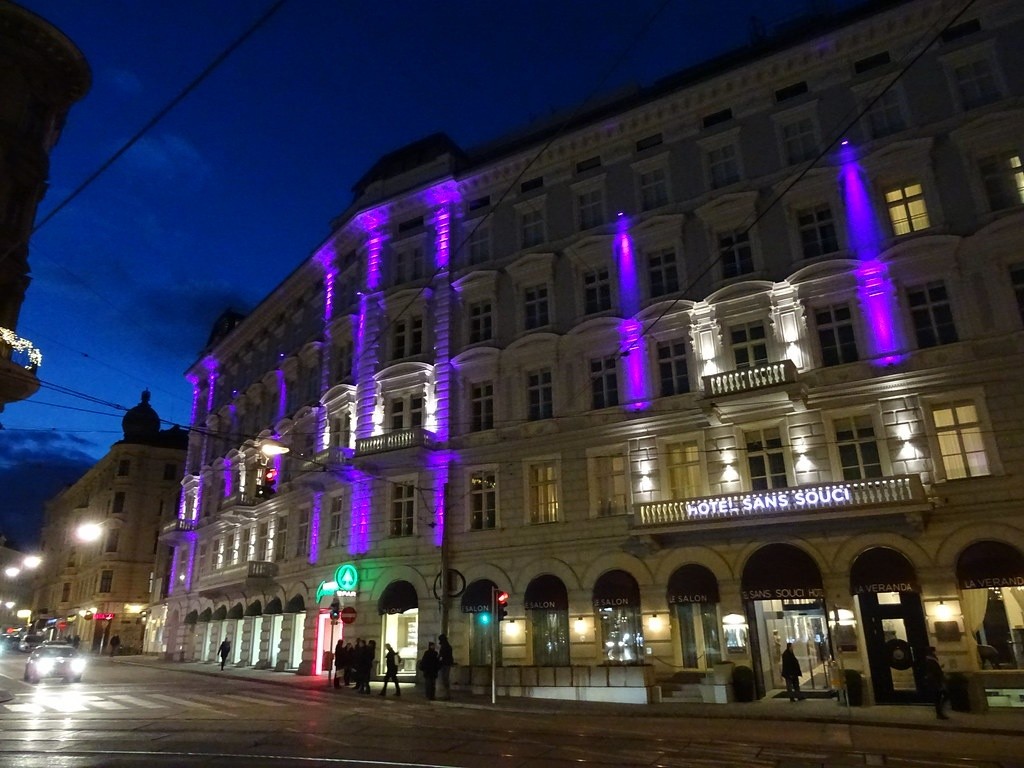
[336,666,345,678]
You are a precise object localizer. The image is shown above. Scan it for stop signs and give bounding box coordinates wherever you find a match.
[58,622,67,630]
[341,607,357,624]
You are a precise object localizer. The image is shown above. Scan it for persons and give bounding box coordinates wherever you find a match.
[334,634,454,701]
[110,634,120,657]
[926,647,949,720]
[64,634,80,650]
[217,638,230,671]
[781,643,806,702]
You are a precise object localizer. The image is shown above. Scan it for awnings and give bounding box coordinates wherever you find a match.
[183,541,1024,624]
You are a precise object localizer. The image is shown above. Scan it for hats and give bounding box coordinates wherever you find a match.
[429,642,434,646]
[439,633,447,641]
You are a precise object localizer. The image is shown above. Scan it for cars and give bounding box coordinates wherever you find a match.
[0,633,46,653]
[24,639,86,685]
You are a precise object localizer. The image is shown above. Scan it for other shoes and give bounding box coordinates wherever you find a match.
[799,697,802,700]
[790,699,796,703]
[393,693,401,697]
[359,690,364,694]
[353,686,358,689]
[366,691,370,694]
[379,693,385,696]
[428,695,434,700]
[936,713,948,719]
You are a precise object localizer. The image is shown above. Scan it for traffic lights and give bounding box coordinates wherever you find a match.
[497,590,508,621]
[332,602,339,619]
